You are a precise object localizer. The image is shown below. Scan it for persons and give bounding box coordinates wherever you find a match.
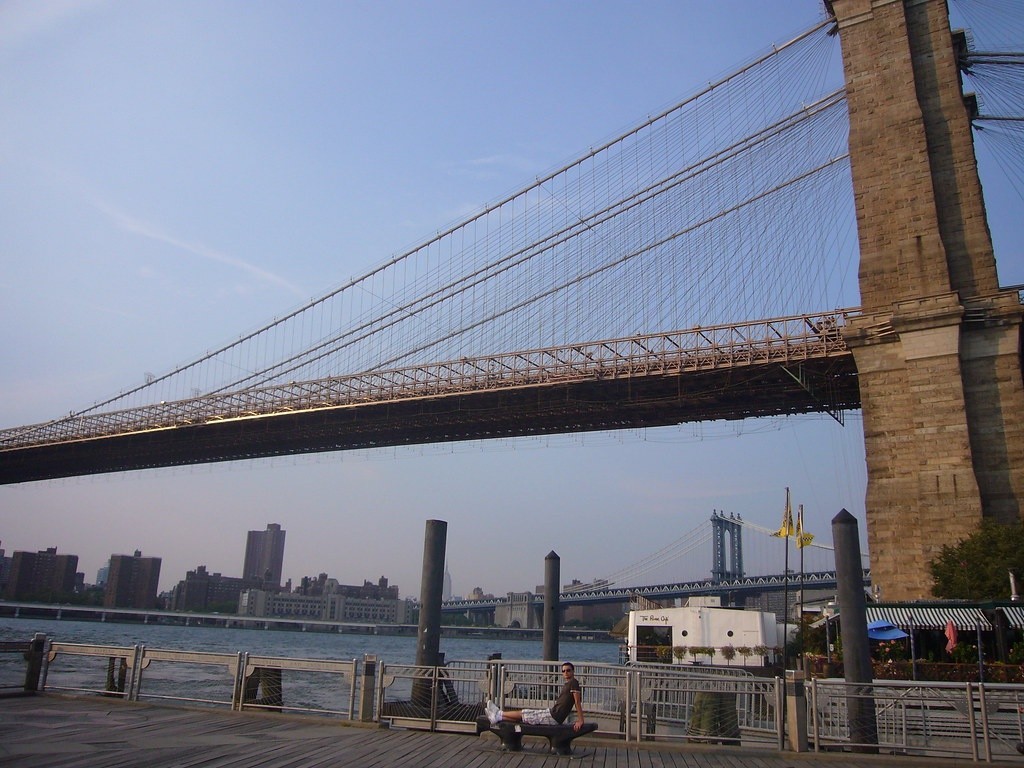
[484,662,584,731]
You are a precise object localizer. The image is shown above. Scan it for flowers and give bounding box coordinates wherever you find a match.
[876,639,904,661]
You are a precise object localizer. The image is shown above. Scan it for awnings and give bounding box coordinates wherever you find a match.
[865,609,992,631]
[867,620,894,630]
[868,627,909,640]
[1002,607,1024,628]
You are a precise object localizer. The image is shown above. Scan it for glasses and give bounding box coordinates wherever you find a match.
[562,669,571,672]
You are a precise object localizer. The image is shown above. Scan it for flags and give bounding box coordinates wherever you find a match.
[771,491,794,536]
[795,507,815,548]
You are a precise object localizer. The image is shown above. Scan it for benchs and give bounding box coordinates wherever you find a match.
[475,715,598,757]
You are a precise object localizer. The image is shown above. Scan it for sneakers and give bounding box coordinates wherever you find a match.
[487,700,500,714]
[485,708,498,724]
